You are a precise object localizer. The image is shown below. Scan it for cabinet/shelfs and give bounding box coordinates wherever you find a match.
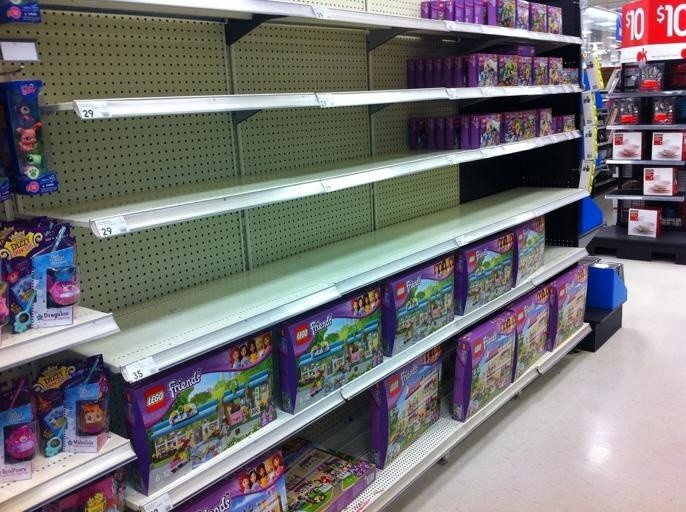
[588,56,686,265]
[0,41,139,507]
[0,3,626,506]
[589,65,622,200]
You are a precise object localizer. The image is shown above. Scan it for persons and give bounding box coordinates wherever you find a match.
[171,447,181,462]
[374,290,381,307]
[368,292,377,311]
[239,343,251,368]
[422,344,442,364]
[500,315,517,335]
[246,339,259,364]
[357,297,366,316]
[265,456,278,485]
[228,346,241,369]
[351,299,361,316]
[362,295,373,314]
[271,453,285,480]
[255,336,266,360]
[238,473,252,495]
[249,467,263,493]
[257,463,273,490]
[183,439,197,461]
[434,257,454,278]
[536,287,550,304]
[497,234,515,252]
[263,333,272,353]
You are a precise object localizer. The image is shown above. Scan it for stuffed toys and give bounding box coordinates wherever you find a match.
[7,425,36,462]
[16,119,44,150]
[50,280,80,308]
[22,153,44,181]
[77,405,106,435]
